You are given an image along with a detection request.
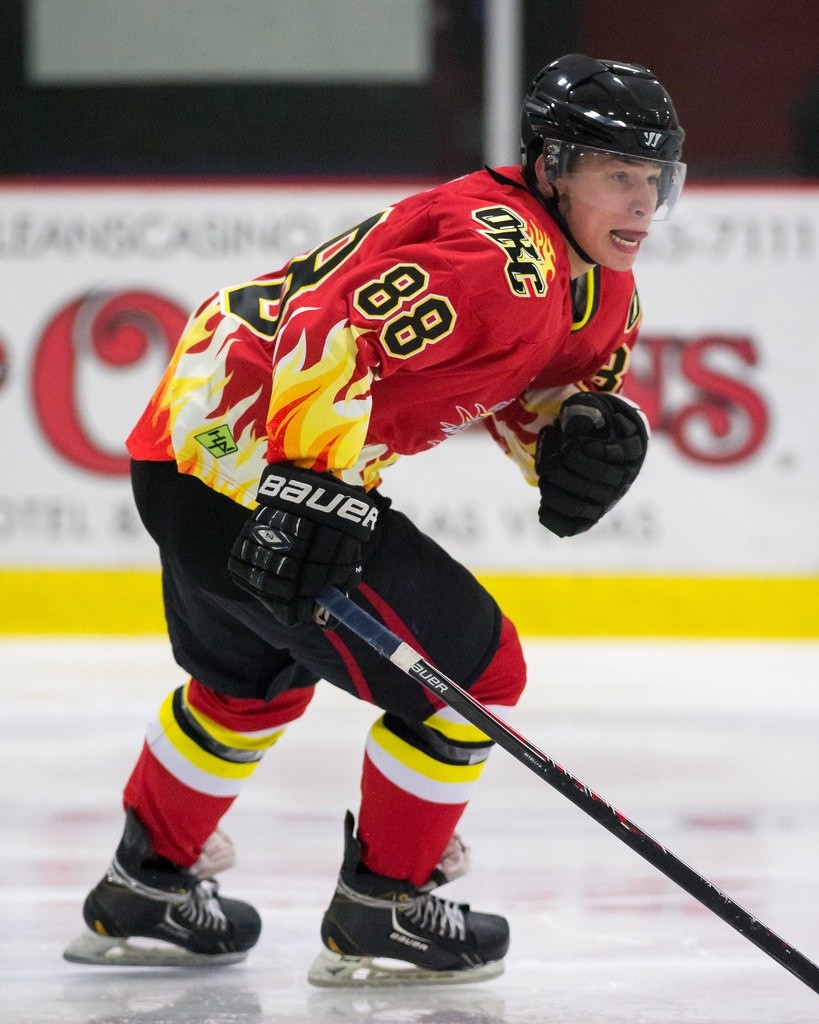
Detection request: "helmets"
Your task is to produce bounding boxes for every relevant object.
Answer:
[520,55,685,164]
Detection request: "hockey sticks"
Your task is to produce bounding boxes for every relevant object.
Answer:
[315,582,819,997]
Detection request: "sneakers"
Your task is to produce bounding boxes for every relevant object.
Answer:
[305,810,511,987]
[59,807,262,969]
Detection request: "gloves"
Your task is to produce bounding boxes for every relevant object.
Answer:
[225,465,392,629]
[534,390,651,539]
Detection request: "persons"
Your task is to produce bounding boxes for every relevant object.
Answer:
[63,50,691,986]
